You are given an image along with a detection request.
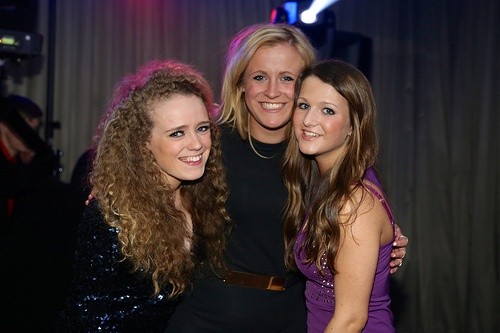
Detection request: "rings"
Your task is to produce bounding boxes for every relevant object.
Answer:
[395,259,403,267]
[400,235,408,246]
[399,249,405,259]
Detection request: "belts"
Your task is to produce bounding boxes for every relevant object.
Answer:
[198,262,291,292]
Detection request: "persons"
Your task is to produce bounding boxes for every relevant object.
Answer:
[85,25,409,333]
[0,57,236,333]
